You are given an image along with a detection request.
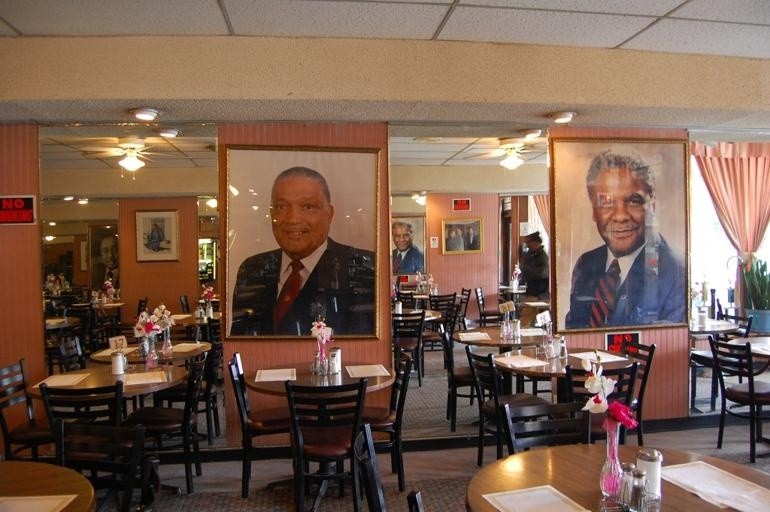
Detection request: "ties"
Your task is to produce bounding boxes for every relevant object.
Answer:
[270,260,306,336]
[589,259,622,328]
[106,271,114,288]
[393,252,402,275]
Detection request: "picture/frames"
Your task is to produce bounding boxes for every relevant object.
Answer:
[393,212,428,277]
[133,207,182,264]
[219,142,384,343]
[549,135,694,336]
[440,215,484,257]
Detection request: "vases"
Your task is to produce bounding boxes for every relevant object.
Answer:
[599,417,624,498]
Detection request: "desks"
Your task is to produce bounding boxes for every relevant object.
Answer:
[466,445,770,512]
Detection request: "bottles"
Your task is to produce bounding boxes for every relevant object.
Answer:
[532,344,542,357]
[637,446,663,505]
[416,271,434,292]
[541,359,570,373]
[193,306,205,319]
[498,318,521,338]
[395,301,403,314]
[545,341,554,358]
[616,463,648,512]
[699,328,706,331]
[313,348,341,375]
[92,294,107,304]
[112,375,125,385]
[698,310,707,328]
[314,376,342,387]
[195,318,206,323]
[427,283,438,294]
[47,273,69,294]
[500,339,521,345]
[391,277,399,296]
[557,340,568,359]
[110,352,129,375]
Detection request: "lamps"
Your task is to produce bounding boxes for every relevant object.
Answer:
[130,103,161,123]
[118,150,145,173]
[549,108,577,126]
[158,126,180,138]
[500,151,525,170]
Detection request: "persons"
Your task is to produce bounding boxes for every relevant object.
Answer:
[230,166,376,335]
[392,222,423,274]
[512,231,549,297]
[93,236,118,291]
[150,223,164,251]
[565,146,687,330]
[446,227,480,251]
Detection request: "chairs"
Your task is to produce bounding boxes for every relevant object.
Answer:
[0,254,225,510]
[228,349,423,511]
[392,279,458,387]
[441,285,657,467]
[689,289,770,463]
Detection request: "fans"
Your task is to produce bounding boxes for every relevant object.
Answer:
[455,134,550,161]
[78,137,175,163]
